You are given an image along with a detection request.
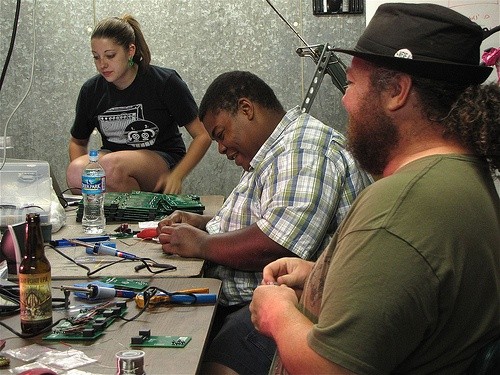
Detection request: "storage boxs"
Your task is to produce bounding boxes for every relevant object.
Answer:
[0,163,52,224]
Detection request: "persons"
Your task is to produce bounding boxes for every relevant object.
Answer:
[248,3,500,375]
[156,71,374,375]
[66,16,212,196]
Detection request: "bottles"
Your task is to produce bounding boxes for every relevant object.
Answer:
[19,213,52,333]
[82,150,105,233]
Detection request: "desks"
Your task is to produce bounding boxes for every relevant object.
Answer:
[0,278,224,375]
[0,195,226,276]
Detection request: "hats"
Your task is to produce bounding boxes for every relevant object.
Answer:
[329,3,494,85]
